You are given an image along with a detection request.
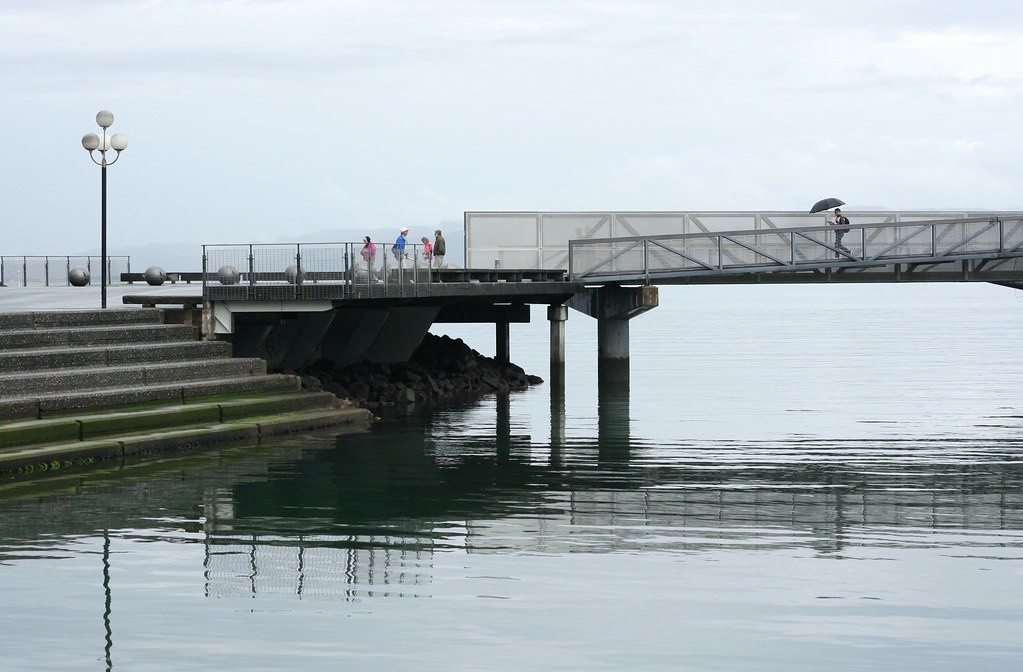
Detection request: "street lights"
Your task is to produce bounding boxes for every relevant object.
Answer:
[82,109,128,309]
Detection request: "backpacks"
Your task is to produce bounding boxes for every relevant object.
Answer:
[840,217,850,232]
[392,244,399,254]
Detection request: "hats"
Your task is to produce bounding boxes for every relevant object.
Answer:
[401,228,410,233]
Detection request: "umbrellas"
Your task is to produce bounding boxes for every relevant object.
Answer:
[809,196,845,224]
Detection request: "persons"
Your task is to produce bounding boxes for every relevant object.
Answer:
[432,229,445,270]
[421,236,433,269]
[826,208,853,260]
[390,227,412,282]
[361,236,379,281]
